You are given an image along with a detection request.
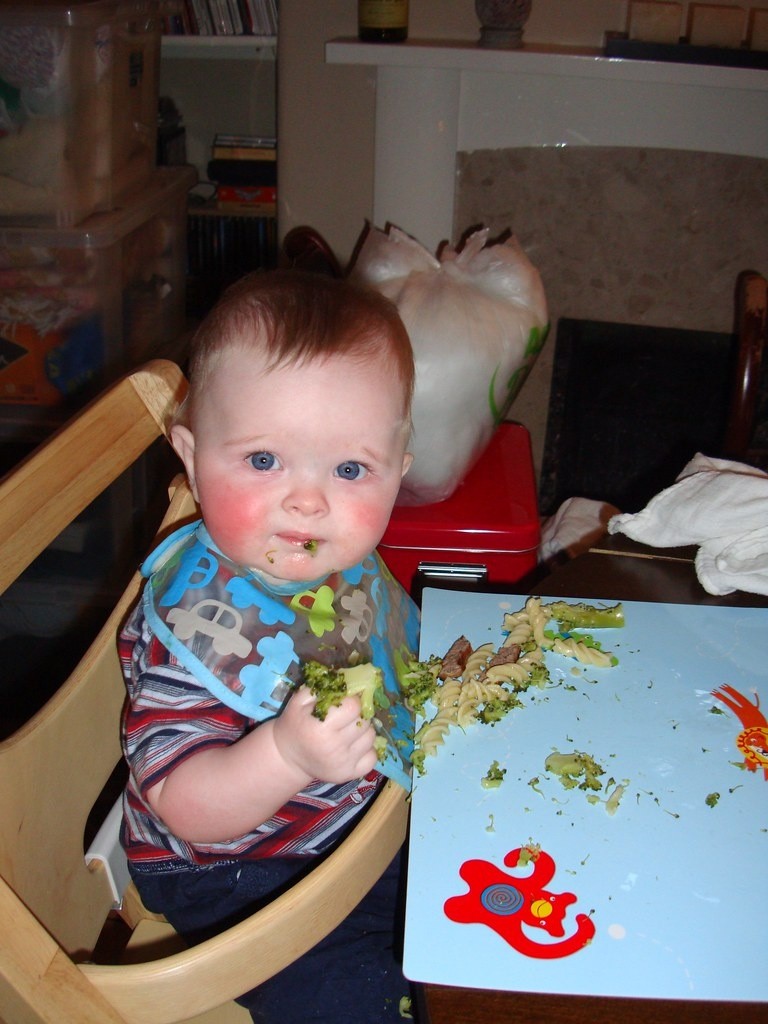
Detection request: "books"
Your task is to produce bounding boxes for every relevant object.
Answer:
[206,133,278,218]
[160,0,278,36]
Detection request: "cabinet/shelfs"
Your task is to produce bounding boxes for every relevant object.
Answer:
[158,34,279,216]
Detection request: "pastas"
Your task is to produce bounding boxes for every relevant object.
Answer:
[420,596,613,757]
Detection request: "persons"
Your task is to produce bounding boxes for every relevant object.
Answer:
[114,253,424,1024]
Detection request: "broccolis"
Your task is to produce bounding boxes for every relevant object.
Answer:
[303,539,626,793]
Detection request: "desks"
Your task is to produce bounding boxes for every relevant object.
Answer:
[1,165,200,509]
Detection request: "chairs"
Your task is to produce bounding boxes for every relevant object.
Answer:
[0,358,411,1024]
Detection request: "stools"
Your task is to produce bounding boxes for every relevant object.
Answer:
[0,391,143,577]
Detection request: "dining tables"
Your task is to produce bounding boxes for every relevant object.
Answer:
[402,531,768,1024]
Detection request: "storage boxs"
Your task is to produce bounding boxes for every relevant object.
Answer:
[0,268,107,406]
[0,0,157,215]
[377,422,541,611]
[212,138,277,185]
[215,184,278,216]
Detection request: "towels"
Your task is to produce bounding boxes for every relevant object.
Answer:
[536,452,768,598]
